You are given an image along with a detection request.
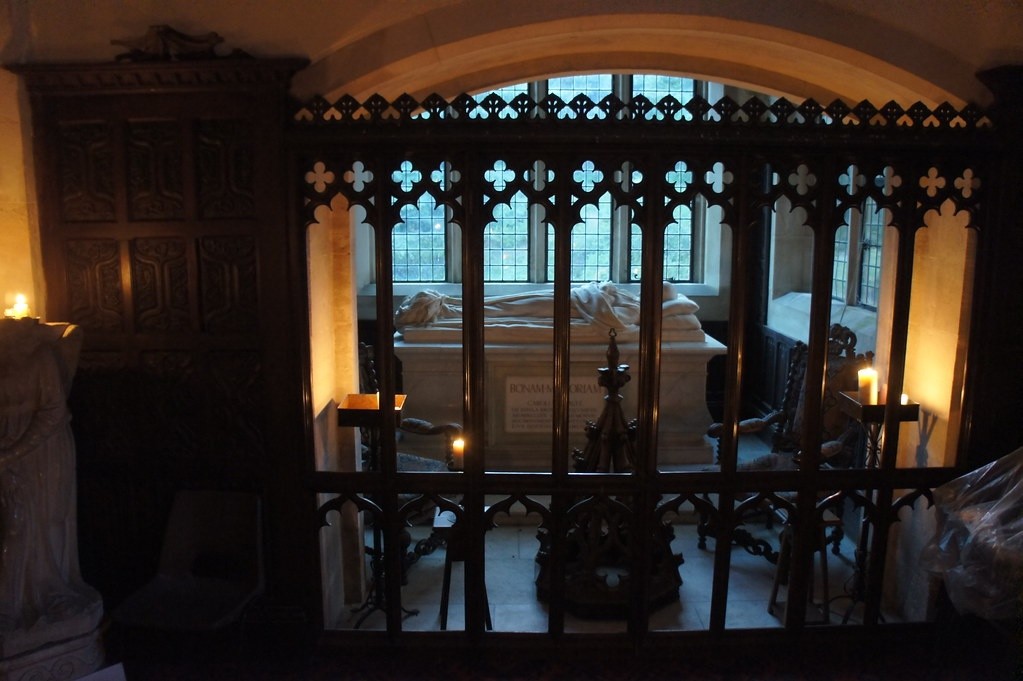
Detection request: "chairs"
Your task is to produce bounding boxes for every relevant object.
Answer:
[696,324,874,586]
[358,340,464,609]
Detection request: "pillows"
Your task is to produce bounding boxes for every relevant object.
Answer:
[661,292,700,317]
[661,314,702,330]
[662,329,706,342]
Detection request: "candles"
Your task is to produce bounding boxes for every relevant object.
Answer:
[13,294,28,316]
[857,368,878,404]
